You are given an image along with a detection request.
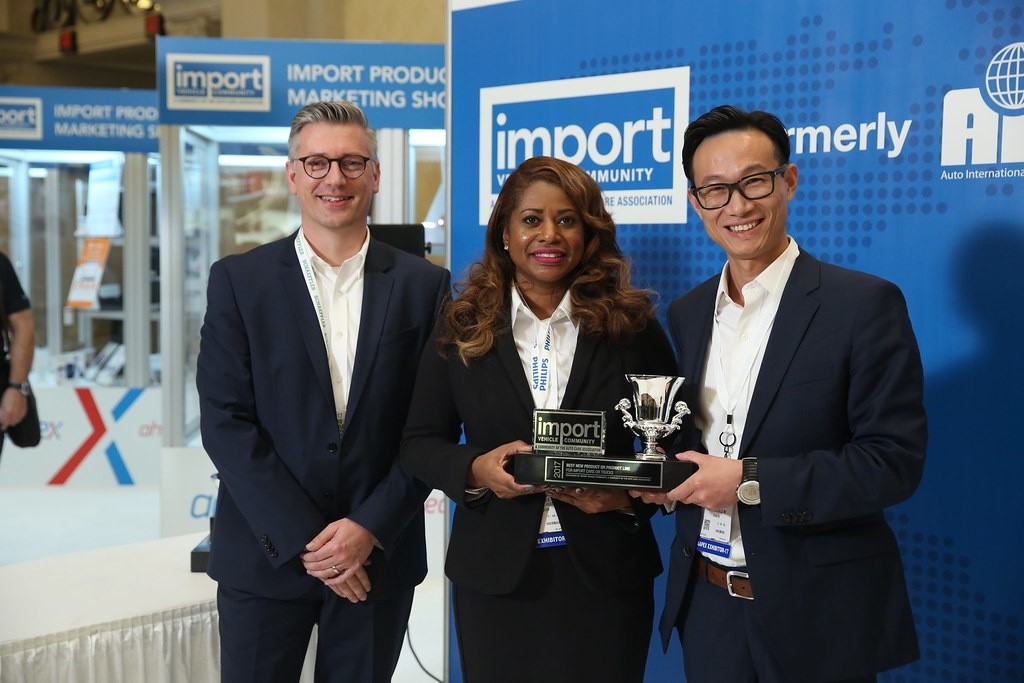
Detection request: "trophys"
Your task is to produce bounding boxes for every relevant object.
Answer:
[513,374,694,493]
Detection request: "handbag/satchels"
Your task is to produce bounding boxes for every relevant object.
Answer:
[4,319,41,447]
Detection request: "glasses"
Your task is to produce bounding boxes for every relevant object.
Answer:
[291,155,377,179]
[691,163,788,210]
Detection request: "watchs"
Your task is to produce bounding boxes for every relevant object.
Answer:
[736,456,761,506]
[7,383,30,396]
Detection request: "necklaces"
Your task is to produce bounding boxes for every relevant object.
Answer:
[515,282,530,310]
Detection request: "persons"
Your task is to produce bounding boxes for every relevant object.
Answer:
[0,251,35,455]
[629,106,928,683]
[397,156,678,683]
[197,100,452,683]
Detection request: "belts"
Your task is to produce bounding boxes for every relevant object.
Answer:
[692,556,756,600]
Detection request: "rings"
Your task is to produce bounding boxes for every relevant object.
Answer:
[333,566,340,574]
